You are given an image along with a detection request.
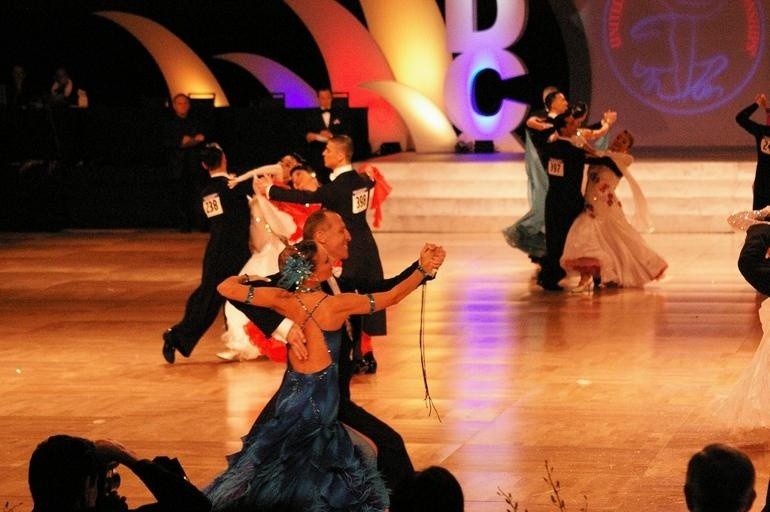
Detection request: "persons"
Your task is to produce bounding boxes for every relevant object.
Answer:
[526,86,635,291]
[301,89,348,179]
[228,211,447,484]
[161,95,211,235]
[163,135,388,375]
[393,466,463,512]
[205,240,439,511]
[684,443,757,512]
[727,93,770,297]
[7,65,31,105]
[51,61,74,108]
[28,435,212,512]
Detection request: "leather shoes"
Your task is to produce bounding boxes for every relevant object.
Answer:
[162,328,175,363]
[216,349,243,362]
[350,356,377,374]
[537,278,564,291]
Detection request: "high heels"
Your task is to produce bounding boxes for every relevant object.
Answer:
[572,274,594,292]
